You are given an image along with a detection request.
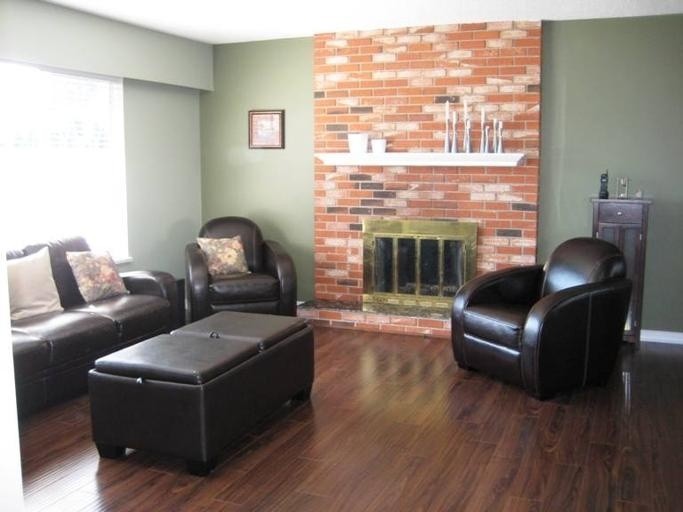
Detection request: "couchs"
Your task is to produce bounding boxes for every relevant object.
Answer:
[452,237,634,401]
[6,237,179,420]
[185,217,297,324]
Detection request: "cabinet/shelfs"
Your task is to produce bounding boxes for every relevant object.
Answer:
[589,197,653,351]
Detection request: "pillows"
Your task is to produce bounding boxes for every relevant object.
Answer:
[7,246,64,322]
[196,234,252,280]
[65,252,130,302]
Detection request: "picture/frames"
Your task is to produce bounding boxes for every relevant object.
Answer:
[248,109,285,150]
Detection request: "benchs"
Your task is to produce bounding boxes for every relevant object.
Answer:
[88,310,315,478]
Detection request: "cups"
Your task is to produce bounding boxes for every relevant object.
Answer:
[347,133,367,155]
[370,139,385,160]
